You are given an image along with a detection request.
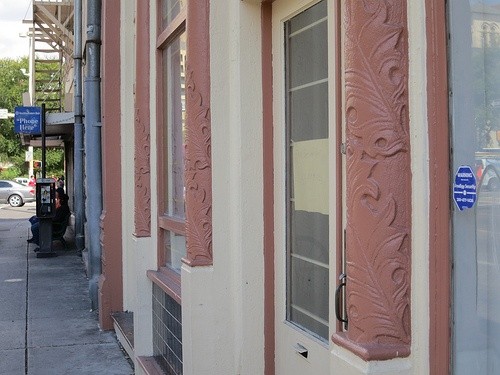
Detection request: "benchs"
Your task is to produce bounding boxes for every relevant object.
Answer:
[33,212,71,253]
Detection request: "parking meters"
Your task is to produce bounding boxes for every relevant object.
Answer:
[34,178,57,257]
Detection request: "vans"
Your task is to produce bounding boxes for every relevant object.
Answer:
[14,178,36,187]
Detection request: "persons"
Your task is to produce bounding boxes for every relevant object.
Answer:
[31,194,71,252]
[27,175,65,243]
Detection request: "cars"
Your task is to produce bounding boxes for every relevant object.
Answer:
[0,179,36,207]
